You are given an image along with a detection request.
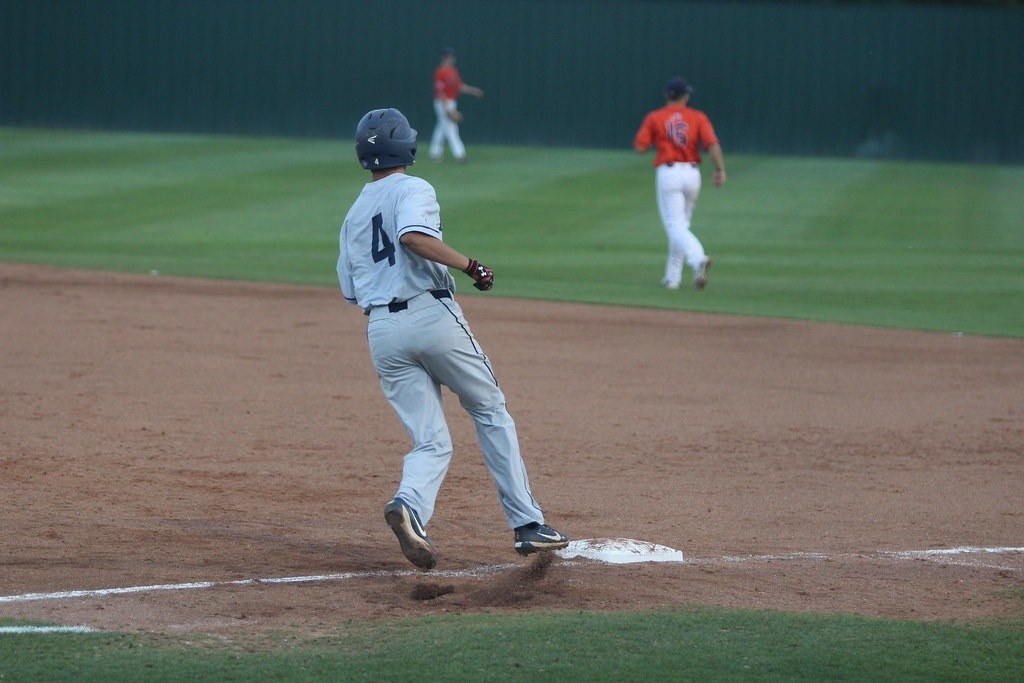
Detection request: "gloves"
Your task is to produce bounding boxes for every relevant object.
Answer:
[463,258,495,291]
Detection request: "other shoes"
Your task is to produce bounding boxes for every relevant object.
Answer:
[696,256,710,288]
[663,279,680,290]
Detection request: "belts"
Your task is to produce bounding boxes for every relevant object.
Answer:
[368,289,452,313]
[668,163,699,168]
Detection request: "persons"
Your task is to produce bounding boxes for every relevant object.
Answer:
[634,79,726,292]
[336,107,571,571]
[428,46,484,166]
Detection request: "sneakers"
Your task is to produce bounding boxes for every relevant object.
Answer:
[514,521,569,554]
[384,498,437,569]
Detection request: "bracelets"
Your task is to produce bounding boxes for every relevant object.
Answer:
[715,168,725,171]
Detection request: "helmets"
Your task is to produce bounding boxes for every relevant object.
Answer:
[355,108,418,169]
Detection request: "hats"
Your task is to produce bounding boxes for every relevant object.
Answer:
[667,77,693,95]
